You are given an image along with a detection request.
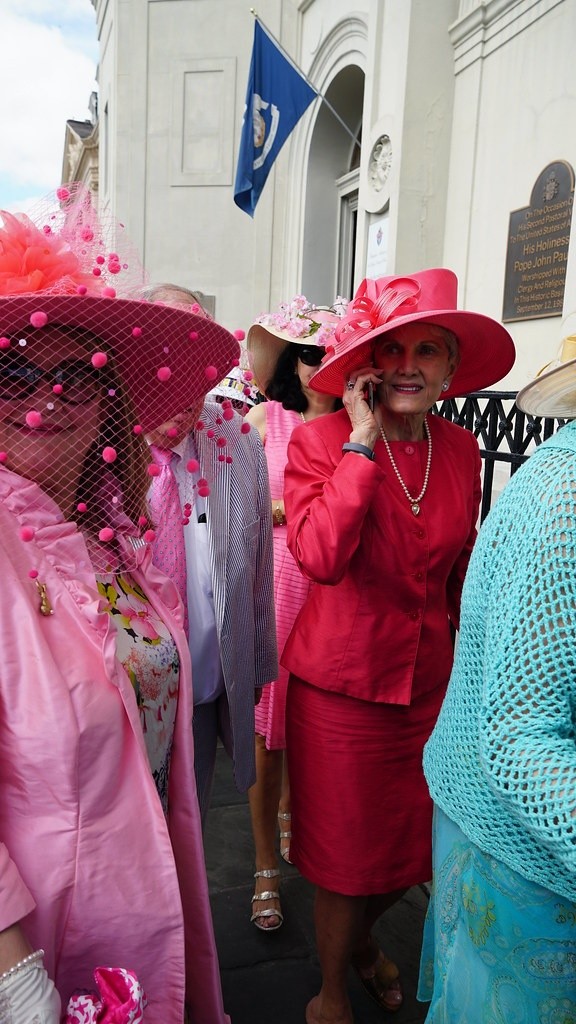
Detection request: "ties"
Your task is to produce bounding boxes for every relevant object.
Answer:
[145,444,190,641]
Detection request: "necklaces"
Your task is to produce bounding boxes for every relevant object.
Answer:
[379,414,433,517]
[300,411,307,423]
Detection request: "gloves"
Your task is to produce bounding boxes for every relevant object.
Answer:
[0,958,62,1024]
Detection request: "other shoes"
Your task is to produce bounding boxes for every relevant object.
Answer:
[349,936,408,1014]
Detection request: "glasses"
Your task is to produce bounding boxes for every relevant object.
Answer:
[215,395,241,406]
[0,350,103,405]
[292,346,325,366]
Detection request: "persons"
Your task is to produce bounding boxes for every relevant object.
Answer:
[205,359,258,417]
[279,266,518,1024]
[236,299,349,935]
[126,283,268,806]
[419,337,576,1024]
[1,181,238,1024]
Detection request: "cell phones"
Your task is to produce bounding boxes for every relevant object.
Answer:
[367,381,374,413]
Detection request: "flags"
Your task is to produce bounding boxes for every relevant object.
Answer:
[233,14,320,221]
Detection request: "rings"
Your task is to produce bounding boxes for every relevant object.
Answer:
[346,380,355,390]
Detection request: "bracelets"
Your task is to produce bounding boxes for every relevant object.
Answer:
[0,947,46,980]
[342,443,376,461]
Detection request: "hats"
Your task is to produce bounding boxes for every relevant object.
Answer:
[0,182,241,434]
[308,268,517,401]
[515,335,576,419]
[245,293,350,401]
[206,328,259,406]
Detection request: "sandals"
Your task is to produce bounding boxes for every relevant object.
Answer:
[276,811,293,865]
[249,869,284,930]
